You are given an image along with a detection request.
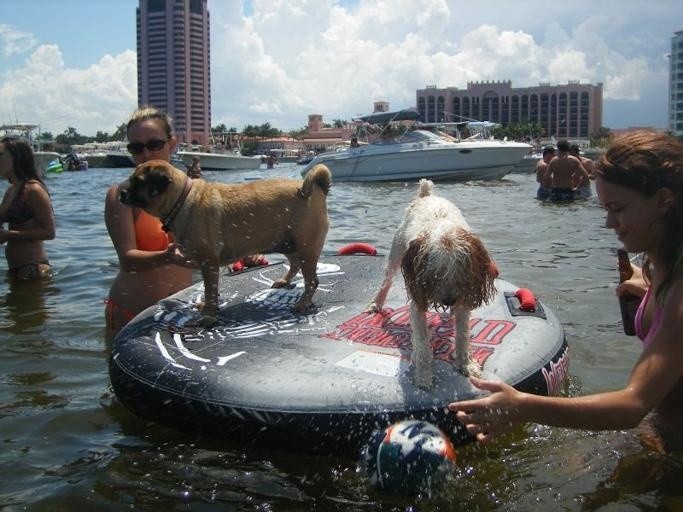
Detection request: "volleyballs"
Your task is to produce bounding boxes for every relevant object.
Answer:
[364,420,457,498]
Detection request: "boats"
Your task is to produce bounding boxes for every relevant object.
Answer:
[0,123,61,178]
[299,104,534,184]
[174,127,265,173]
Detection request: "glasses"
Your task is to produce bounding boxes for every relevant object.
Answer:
[126,139,168,154]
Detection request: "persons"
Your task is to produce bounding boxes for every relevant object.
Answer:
[297,148,301,157]
[267,153,279,169]
[446,128,683,453]
[349,133,360,150]
[102,106,269,337]
[535,145,556,201]
[1,134,58,282]
[568,142,599,201]
[300,148,305,157]
[63,152,89,172]
[544,139,589,204]
[186,156,204,180]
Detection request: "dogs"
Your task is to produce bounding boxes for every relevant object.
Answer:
[117,159,332,317]
[369,178,499,392]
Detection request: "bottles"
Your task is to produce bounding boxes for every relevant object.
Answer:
[616,248,643,337]
